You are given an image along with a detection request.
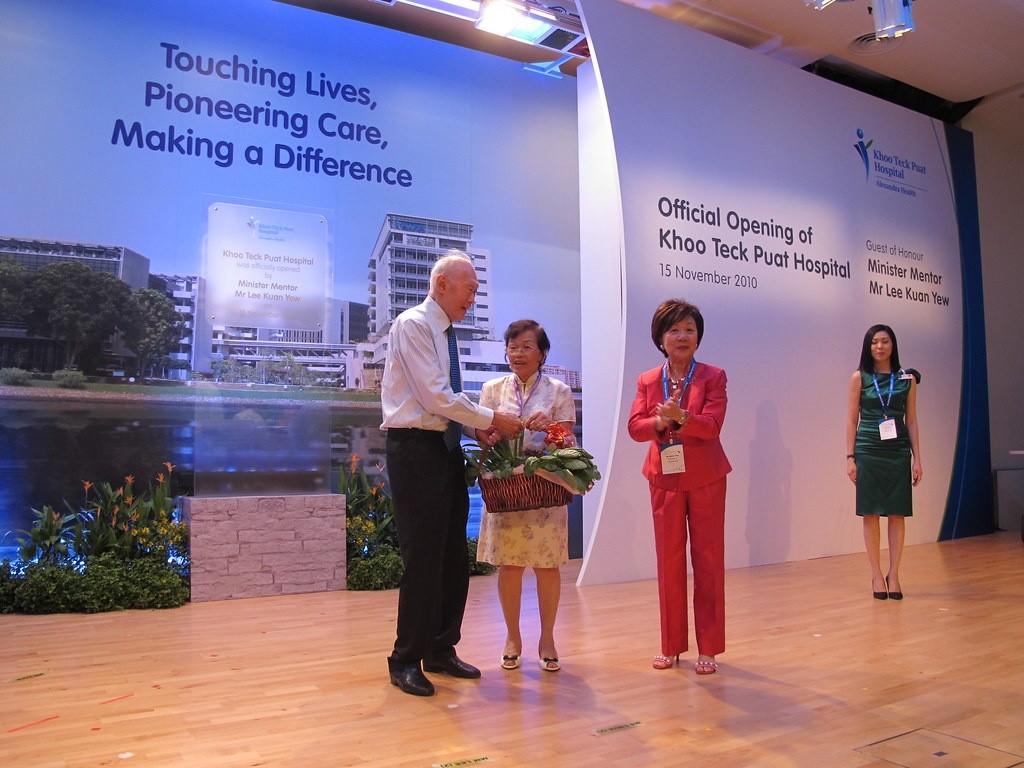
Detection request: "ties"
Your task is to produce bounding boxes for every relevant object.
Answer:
[443,325,462,452]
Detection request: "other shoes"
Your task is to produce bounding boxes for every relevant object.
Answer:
[540,657,561,671]
[501,653,523,669]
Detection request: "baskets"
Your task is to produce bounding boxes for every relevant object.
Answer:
[478,422,573,513]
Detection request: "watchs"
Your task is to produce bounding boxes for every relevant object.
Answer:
[677,410,686,425]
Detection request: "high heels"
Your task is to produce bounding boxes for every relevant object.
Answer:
[696,662,717,674]
[872,579,888,599]
[653,654,680,669]
[885,576,903,600]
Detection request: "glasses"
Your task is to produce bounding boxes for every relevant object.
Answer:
[505,344,540,355]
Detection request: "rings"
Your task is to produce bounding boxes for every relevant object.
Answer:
[539,422,542,425]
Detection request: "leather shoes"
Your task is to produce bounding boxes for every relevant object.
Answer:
[424,653,481,679]
[389,666,434,697]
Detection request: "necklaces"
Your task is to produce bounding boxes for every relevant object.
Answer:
[514,371,542,418]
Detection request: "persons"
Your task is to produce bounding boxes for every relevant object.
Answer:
[847,324,922,600]
[379,249,526,696]
[477,320,575,670]
[628,299,732,674]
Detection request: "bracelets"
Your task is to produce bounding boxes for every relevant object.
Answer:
[847,455,854,459]
